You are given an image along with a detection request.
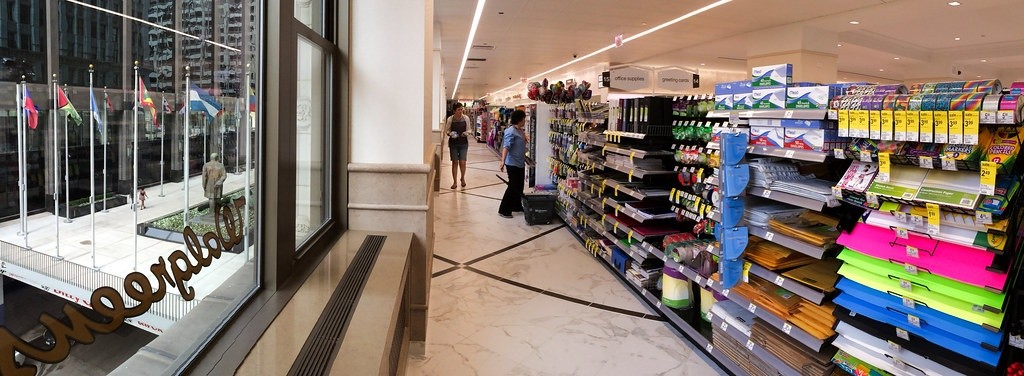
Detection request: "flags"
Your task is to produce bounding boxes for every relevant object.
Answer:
[15,77,257,132]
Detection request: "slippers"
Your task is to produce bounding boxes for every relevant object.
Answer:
[460,179,466,187]
[451,184,457,189]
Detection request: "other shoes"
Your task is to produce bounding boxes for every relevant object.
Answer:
[500,212,513,218]
[513,207,524,212]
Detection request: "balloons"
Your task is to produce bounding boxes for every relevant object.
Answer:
[527,78,592,104]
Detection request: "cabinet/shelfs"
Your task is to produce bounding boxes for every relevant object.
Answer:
[463,103,1024,376]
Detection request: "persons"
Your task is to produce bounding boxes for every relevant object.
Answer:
[446,102,472,189]
[139,187,148,210]
[129,187,134,209]
[498,110,532,218]
[203,152,228,217]
